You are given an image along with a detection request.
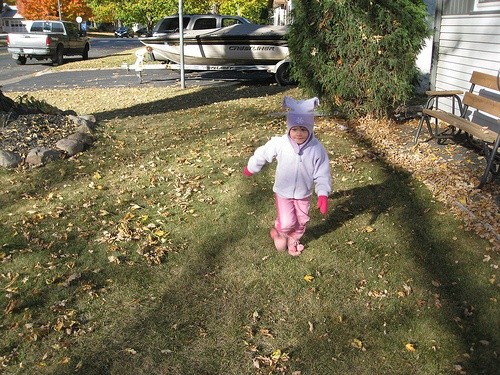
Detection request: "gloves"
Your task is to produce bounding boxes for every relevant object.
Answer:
[316,195,329,214]
[243,167,252,176]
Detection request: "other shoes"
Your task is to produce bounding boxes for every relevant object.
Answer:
[287,237,304,256]
[271,228,288,252]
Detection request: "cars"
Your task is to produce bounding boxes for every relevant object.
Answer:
[113,26,134,38]
[136,28,155,36]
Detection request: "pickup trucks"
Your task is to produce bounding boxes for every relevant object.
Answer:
[6,20,90,66]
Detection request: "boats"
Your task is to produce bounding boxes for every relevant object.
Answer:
[139,23,316,65]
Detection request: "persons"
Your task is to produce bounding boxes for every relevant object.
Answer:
[243,97,332,256]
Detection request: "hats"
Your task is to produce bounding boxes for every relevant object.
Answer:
[283,96,320,154]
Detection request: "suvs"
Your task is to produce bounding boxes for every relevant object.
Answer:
[148,14,254,73]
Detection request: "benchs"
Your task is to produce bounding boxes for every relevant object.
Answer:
[413,70,500,190]
